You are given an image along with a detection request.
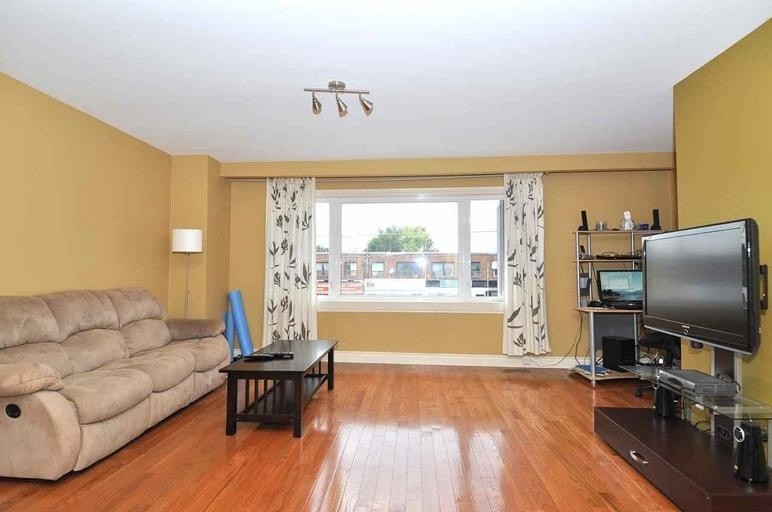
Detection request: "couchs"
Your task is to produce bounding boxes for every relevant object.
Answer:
[0,287,231,482]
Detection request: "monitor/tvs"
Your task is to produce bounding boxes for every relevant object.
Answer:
[597,269,642,303]
[643,219,761,355]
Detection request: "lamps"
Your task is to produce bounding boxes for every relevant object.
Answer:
[304,82,373,114]
[171,229,203,320]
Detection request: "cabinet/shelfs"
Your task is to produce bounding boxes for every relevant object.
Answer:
[573,230,661,308]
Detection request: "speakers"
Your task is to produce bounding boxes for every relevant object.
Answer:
[578,210,588,230]
[651,209,661,230]
[602,335,636,373]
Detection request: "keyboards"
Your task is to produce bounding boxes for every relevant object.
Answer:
[615,303,643,310]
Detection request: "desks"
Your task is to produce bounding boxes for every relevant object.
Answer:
[574,308,643,388]
[594,364,772,512]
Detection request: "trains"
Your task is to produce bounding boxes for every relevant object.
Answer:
[315,252,497,296]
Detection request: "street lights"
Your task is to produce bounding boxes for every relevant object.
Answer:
[168,226,205,316]
